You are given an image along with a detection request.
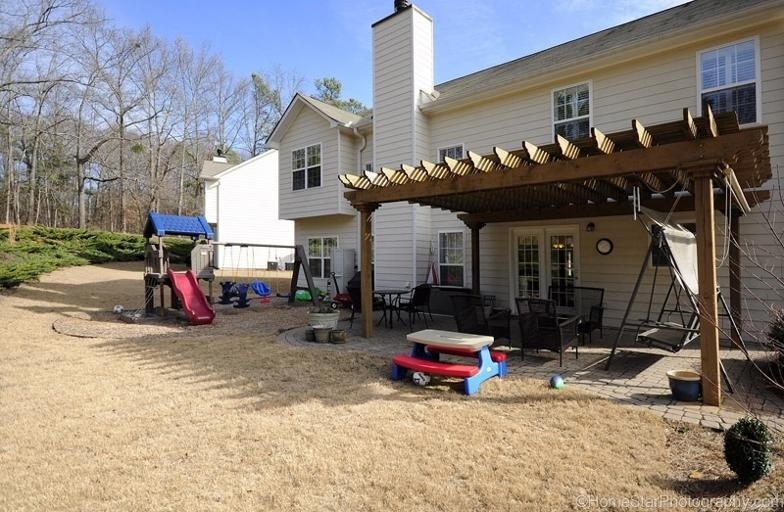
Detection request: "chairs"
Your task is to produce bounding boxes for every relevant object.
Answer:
[514,295,582,371]
[390,283,437,329]
[546,285,605,343]
[346,271,387,331]
[450,290,514,348]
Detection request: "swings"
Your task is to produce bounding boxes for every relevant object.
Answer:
[252,246,272,304]
[234,245,252,308]
[636,230,701,352]
[219,245,238,305]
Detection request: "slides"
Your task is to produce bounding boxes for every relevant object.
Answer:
[167,268,216,325]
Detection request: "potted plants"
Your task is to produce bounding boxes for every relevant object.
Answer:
[724,417,774,482]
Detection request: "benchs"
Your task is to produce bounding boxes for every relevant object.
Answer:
[422,342,508,364]
[634,291,722,355]
[390,355,480,399]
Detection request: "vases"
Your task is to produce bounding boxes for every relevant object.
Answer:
[665,370,701,402]
[304,308,345,343]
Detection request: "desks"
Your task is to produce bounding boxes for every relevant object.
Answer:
[405,326,495,354]
[374,289,412,327]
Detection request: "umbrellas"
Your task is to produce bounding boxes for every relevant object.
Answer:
[425,260,440,285]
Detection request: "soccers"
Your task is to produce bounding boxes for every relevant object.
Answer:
[412,371,431,386]
[114,305,124,314]
